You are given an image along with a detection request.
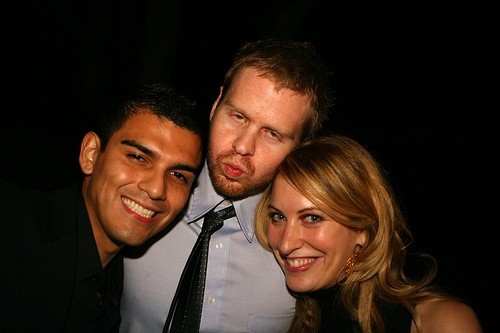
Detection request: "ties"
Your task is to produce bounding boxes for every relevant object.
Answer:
[162,206,236,333]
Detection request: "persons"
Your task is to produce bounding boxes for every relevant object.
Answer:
[115,34,338,333]
[253,130,482,333]
[0,79,210,333]
